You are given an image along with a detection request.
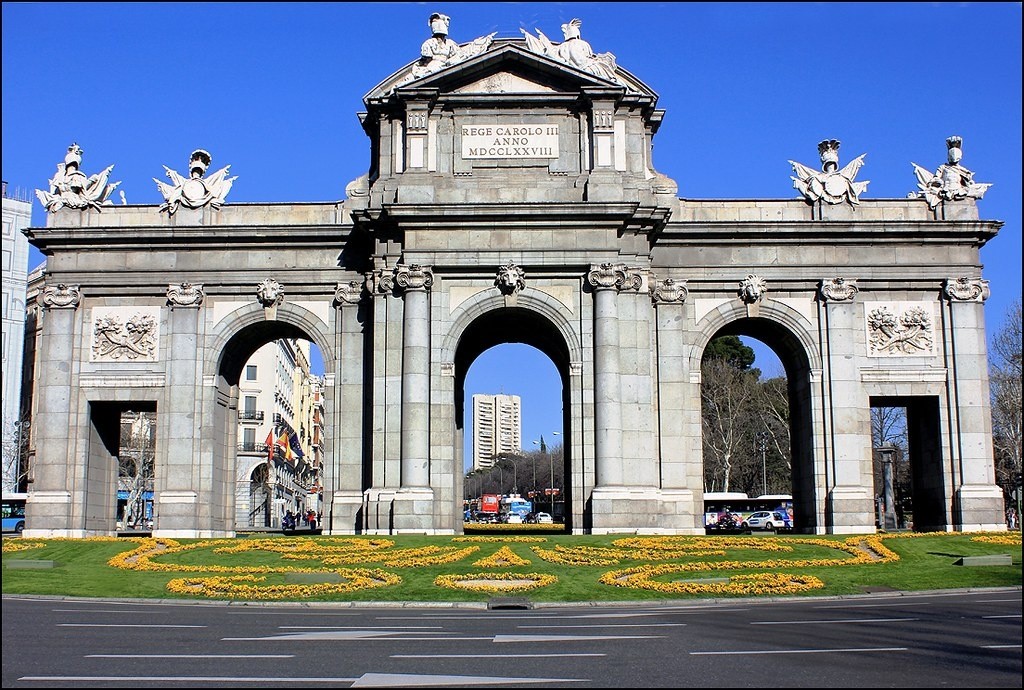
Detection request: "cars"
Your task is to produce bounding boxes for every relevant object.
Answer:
[462,494,534,524]
[740,511,786,530]
[531,511,553,524]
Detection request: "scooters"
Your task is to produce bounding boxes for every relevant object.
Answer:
[281,516,296,531]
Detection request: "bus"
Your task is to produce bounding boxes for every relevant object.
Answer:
[703,492,794,528]
[2,491,154,534]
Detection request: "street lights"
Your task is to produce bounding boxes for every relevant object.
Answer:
[501,457,517,497]
[532,440,554,519]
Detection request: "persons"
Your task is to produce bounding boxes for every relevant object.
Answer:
[465,509,479,522]
[283,509,321,528]
[725,509,732,519]
[1006,508,1018,529]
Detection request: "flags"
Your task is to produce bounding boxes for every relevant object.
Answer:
[265,429,273,460]
[276,429,292,461]
[289,433,305,457]
